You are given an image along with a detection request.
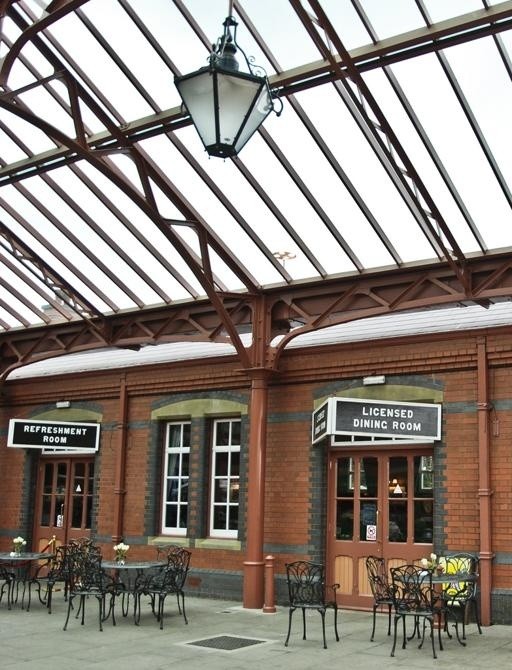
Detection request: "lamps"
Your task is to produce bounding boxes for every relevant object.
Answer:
[172,0,285,164]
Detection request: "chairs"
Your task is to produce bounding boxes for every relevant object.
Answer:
[0,535,193,634]
[364,552,487,660]
[283,559,341,650]
